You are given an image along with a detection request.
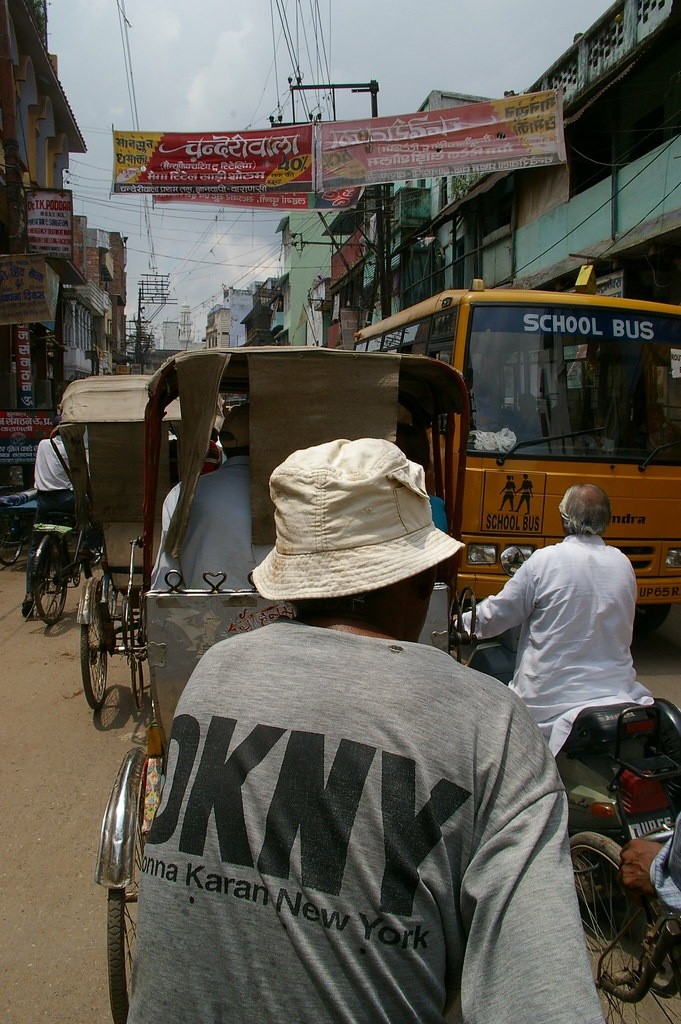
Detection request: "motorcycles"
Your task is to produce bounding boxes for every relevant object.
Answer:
[432,546,680,914]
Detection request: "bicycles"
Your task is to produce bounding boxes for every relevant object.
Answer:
[0,483,34,566]
[29,512,104,625]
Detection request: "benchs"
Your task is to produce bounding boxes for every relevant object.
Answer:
[142,570,449,757]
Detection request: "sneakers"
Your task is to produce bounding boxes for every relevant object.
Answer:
[20,591,33,620]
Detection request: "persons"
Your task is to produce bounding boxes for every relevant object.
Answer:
[396,424,448,536]
[22,415,84,620]
[454,482,654,757]
[146,403,275,590]
[111,438,614,1024]
[467,349,615,454]
[619,811,681,912]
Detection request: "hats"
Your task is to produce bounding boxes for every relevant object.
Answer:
[217,405,249,448]
[252,438,464,600]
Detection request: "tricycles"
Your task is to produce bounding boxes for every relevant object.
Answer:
[49,344,222,712]
[93,349,467,1022]
[567,831,681,1024]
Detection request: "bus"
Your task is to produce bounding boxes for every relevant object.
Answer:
[352,263,681,639]
